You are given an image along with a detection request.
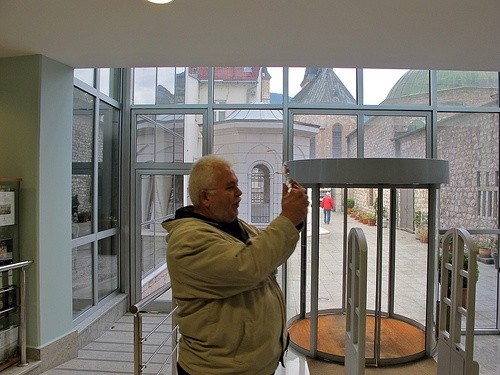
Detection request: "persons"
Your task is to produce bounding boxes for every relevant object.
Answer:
[320,192,334,224]
[161,154,308,375]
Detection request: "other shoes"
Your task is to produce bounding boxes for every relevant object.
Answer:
[324,222,330,224]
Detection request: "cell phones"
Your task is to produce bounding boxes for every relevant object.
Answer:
[283,161,293,188]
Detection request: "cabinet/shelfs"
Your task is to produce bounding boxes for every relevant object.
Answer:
[0,177,22,372]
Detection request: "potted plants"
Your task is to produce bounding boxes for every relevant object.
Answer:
[344,198,388,227]
[437,252,481,309]
[479,241,492,256]
[420,225,428,243]
[492,235,500,270]
[413,212,423,240]
